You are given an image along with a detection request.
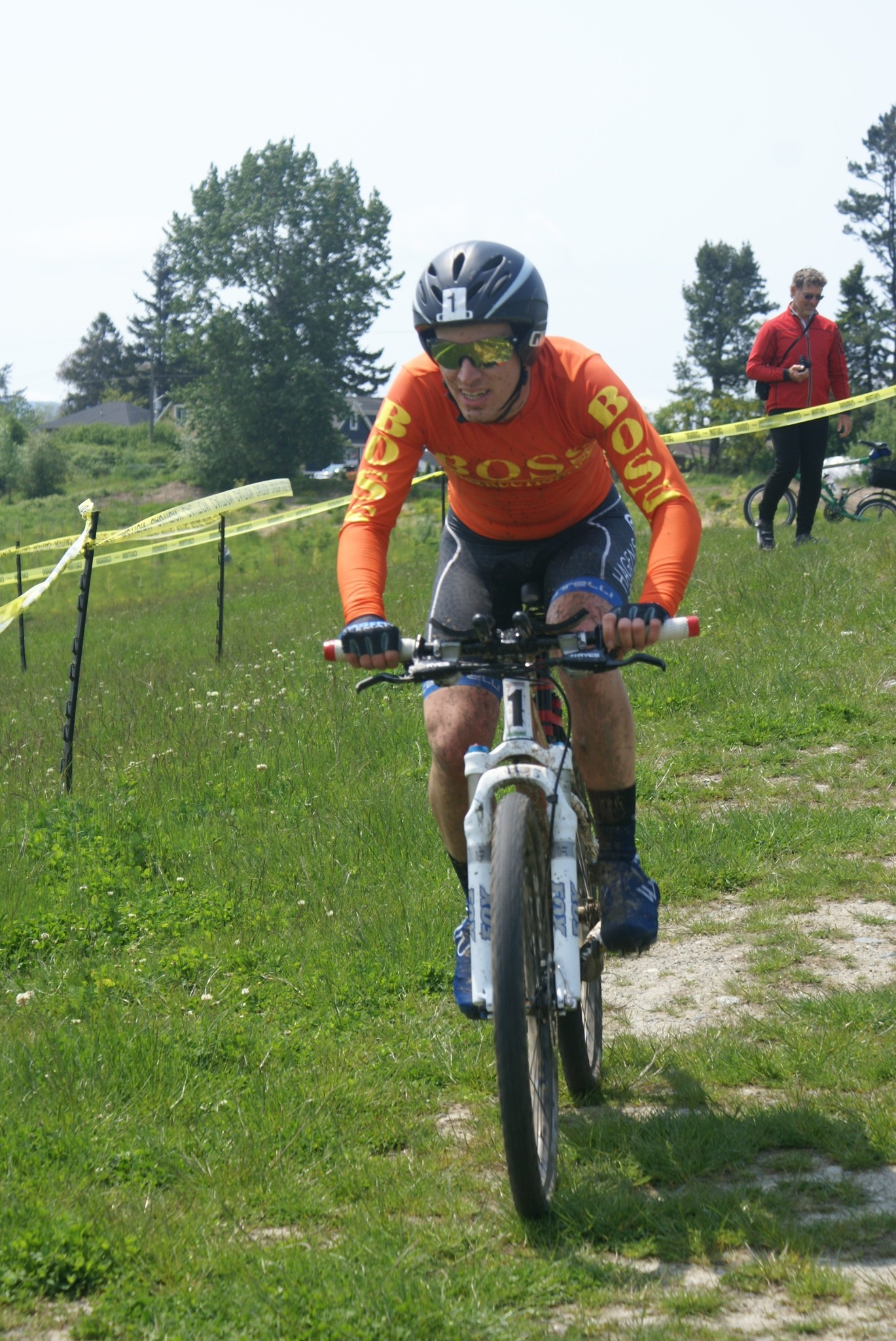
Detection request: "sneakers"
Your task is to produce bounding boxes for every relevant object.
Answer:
[795,533,817,546]
[596,852,661,953]
[755,520,775,550]
[453,916,488,1019]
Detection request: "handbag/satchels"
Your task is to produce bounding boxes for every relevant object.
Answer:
[868,466,896,490]
[755,381,768,400]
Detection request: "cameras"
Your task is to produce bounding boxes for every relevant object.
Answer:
[798,356,811,372]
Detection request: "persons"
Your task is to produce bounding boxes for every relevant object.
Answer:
[335,240,703,1023]
[744,268,854,551]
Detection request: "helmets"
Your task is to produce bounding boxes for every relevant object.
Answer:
[413,240,548,364]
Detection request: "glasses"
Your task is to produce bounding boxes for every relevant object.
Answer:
[424,335,516,369]
[797,287,824,300]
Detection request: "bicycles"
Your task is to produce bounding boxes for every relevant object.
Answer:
[320,573,703,1221]
[742,438,896,530]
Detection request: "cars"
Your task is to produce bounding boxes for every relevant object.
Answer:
[417,448,445,479]
[310,463,357,481]
